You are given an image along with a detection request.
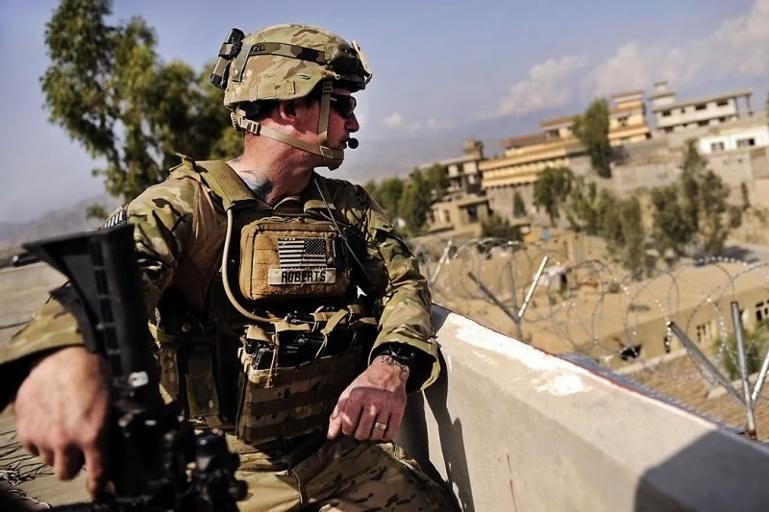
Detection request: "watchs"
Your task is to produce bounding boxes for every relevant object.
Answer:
[372,344,418,368]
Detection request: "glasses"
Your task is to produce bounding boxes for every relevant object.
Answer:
[311,90,357,118]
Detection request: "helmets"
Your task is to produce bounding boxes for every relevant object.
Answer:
[213,23,366,109]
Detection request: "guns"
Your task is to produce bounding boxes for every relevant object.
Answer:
[21,222,249,512]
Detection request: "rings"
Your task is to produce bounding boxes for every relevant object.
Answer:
[375,422,388,429]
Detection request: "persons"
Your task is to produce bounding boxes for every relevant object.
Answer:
[0,24,464,512]
